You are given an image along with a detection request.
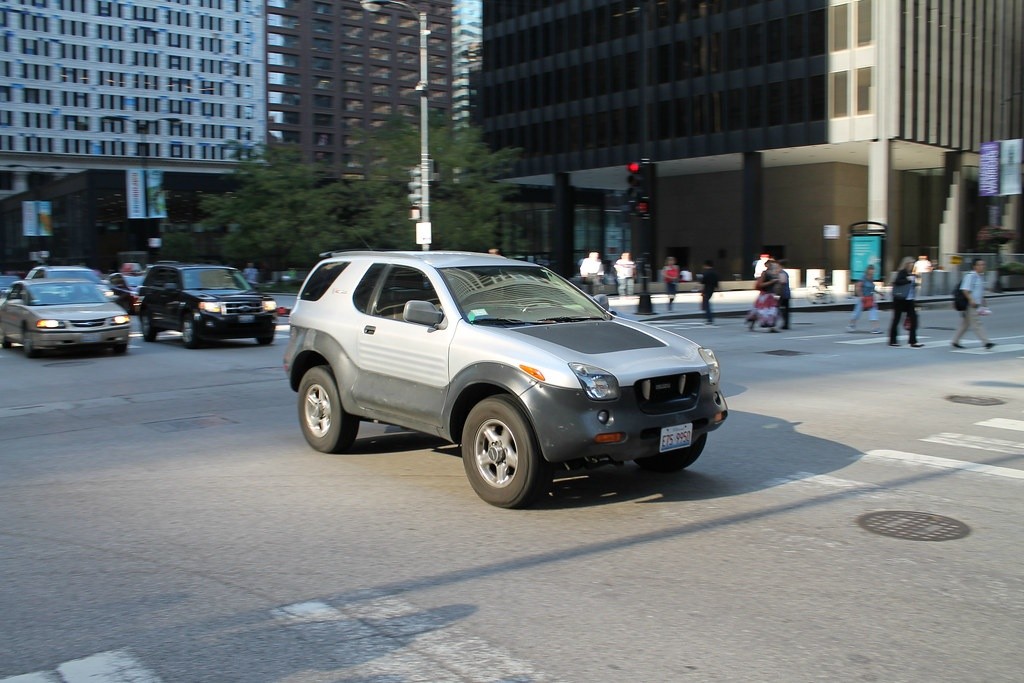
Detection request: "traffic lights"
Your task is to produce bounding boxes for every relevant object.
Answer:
[625,158,649,206]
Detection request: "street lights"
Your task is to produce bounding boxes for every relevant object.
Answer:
[357,0,431,251]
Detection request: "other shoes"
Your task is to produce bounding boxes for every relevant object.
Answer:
[844,326,856,333]
[951,342,964,350]
[870,329,882,334]
[911,343,925,349]
[985,343,997,350]
[889,343,903,346]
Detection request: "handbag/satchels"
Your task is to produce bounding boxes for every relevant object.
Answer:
[892,281,911,299]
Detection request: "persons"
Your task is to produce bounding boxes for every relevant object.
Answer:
[243,263,259,286]
[580,251,605,294]
[847,265,884,335]
[888,255,924,347]
[952,258,995,349]
[662,256,680,311]
[912,254,942,295]
[699,260,719,325]
[678,269,693,282]
[746,261,791,331]
[614,253,637,295]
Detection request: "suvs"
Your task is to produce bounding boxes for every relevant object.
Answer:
[137,260,281,347]
[283,250,730,510]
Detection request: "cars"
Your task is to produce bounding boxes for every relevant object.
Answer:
[0,276,23,308]
[21,265,119,302]
[0,277,132,360]
[106,270,145,314]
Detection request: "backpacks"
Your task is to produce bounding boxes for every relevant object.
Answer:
[952,279,969,312]
